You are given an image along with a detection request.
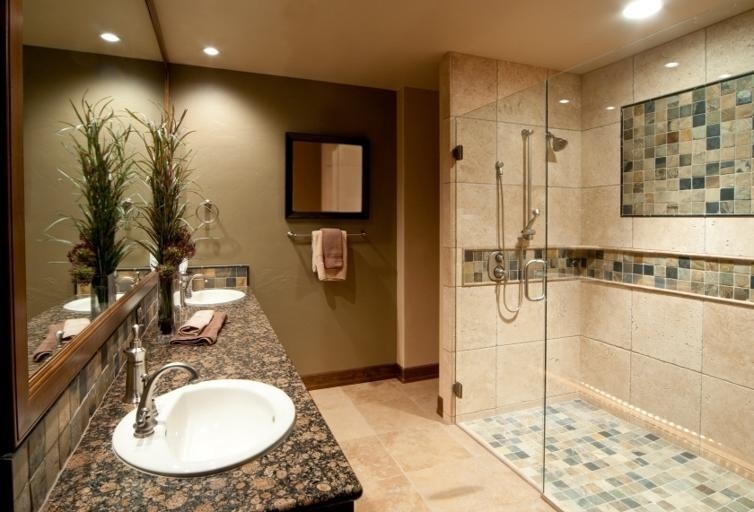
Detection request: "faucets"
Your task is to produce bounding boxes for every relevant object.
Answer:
[136,360,196,421]
[178,273,203,298]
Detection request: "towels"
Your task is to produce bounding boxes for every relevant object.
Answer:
[170,310,226,347]
[30,319,87,370]
[311,227,348,282]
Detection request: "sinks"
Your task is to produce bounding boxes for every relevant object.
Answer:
[63,292,126,314]
[111,379,297,474]
[173,288,247,306]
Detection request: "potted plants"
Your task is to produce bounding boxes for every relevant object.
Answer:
[46,88,142,322]
[126,107,204,334]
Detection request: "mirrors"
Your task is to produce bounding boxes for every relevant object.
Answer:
[285,130,370,220]
[2,0,173,453]
